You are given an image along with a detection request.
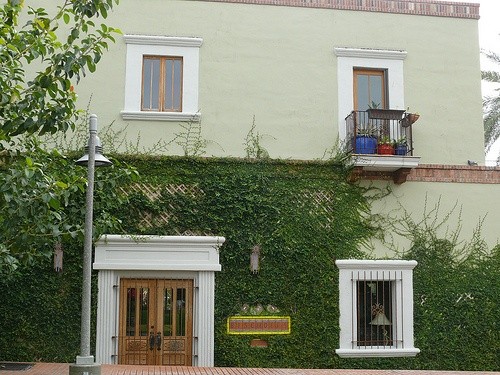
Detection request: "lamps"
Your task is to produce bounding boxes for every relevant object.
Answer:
[368,312,393,345]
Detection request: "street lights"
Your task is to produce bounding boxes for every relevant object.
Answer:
[72,114,113,364]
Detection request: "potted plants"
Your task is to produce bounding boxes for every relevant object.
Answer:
[356,124,408,156]
